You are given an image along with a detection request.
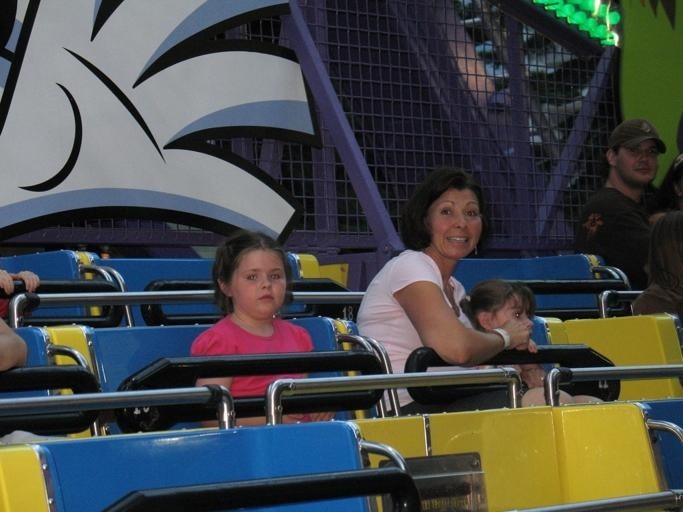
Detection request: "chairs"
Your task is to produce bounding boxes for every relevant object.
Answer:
[0,247,683,512]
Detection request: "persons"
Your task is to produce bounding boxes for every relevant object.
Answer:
[0,314,26,372]
[628,208,682,329]
[0,267,42,328]
[574,117,670,292]
[188,229,340,428]
[354,163,608,417]
[652,152,682,210]
[458,278,549,390]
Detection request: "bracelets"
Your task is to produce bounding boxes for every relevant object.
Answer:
[489,325,511,353]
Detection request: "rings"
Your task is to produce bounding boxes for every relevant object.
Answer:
[538,374,545,381]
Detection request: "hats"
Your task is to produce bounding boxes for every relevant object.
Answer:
[607,118,667,154]
